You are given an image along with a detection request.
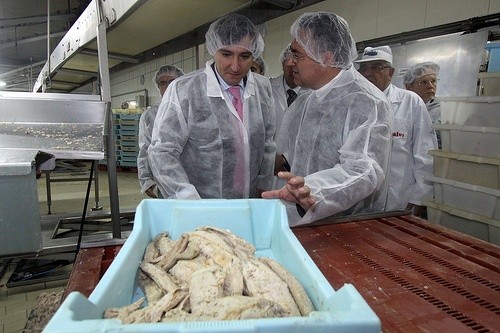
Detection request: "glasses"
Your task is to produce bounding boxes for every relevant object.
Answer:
[415,78,440,86]
[157,80,168,89]
[356,65,390,74]
[289,49,307,62]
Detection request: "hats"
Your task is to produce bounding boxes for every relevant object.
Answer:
[353,45,393,64]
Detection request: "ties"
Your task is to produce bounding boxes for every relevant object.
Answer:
[286,89,297,108]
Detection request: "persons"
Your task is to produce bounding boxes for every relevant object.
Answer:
[137,46,442,212]
[147,15,276,199]
[262,11,391,227]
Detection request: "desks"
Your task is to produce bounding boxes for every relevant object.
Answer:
[58,212,500,333]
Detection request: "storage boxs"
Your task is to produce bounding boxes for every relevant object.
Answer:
[425,176,500,221]
[433,124,500,155]
[485,42,500,72]
[112,108,146,168]
[427,150,500,191]
[422,199,500,246]
[435,96,500,129]
[38,199,382,333]
[478,72,500,96]
